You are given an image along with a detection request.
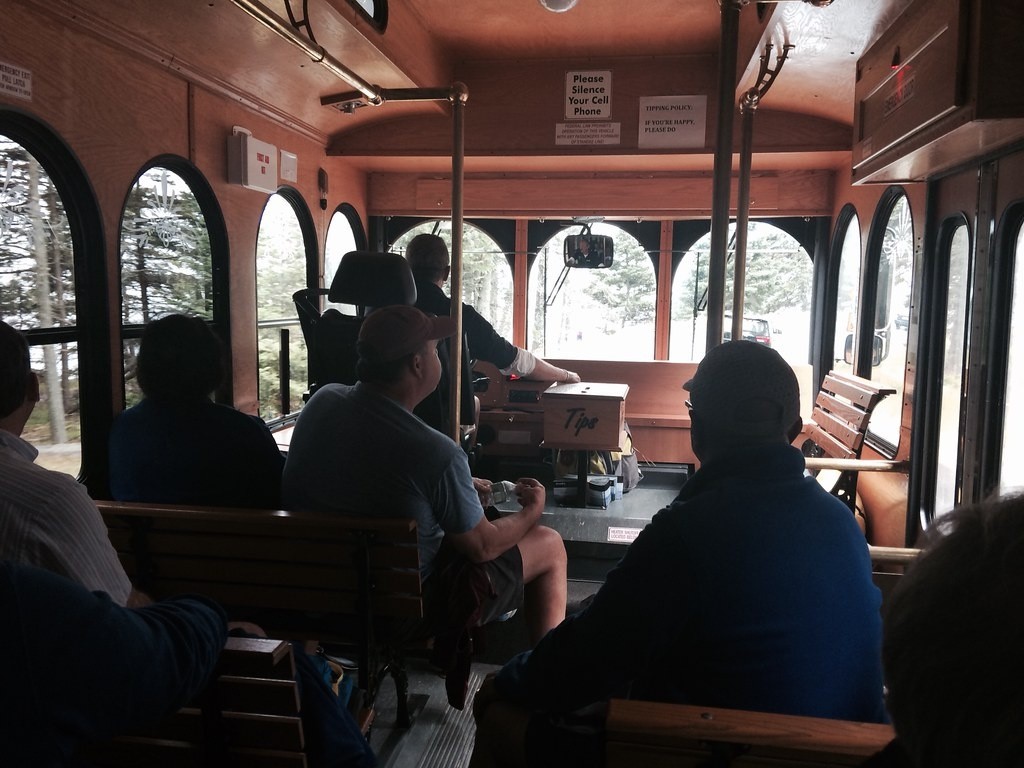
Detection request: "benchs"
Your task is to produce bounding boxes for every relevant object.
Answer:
[604,547,940,768]
[0,497,502,768]
[796,366,898,516]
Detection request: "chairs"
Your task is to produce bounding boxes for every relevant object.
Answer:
[307,250,476,462]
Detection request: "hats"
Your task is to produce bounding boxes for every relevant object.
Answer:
[357,305,458,362]
[684,340,800,436]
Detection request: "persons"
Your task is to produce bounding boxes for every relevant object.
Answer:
[0,558,230,768]
[467,341,886,768]
[407,234,580,455]
[854,491,1024,768]
[0,322,265,637]
[280,304,569,643]
[566,238,606,267]
[102,313,287,511]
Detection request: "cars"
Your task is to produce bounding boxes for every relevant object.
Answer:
[724,314,782,350]
[895,308,909,330]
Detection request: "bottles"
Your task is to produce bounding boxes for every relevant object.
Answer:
[477,480,517,508]
